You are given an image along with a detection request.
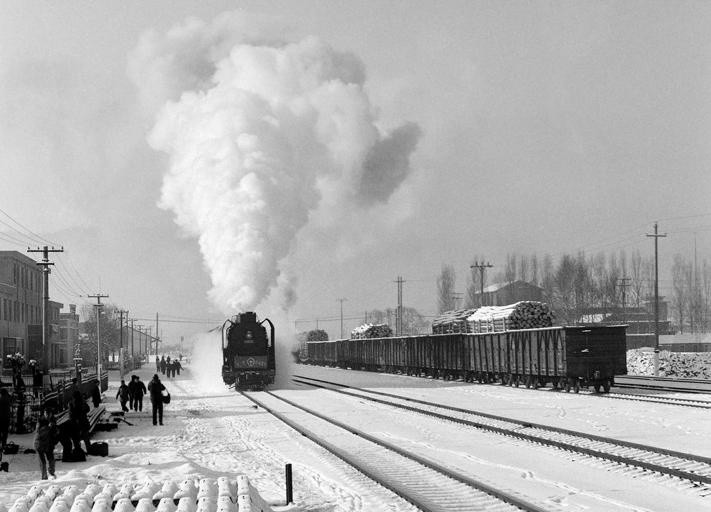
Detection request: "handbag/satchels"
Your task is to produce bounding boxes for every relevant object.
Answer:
[4,443,18,455]
[162,394,170,404]
[92,441,108,455]
[63,447,86,462]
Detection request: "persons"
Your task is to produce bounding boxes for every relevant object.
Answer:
[0,355,185,479]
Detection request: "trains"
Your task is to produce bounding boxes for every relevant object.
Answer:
[288,323,631,394]
[187,311,275,394]
